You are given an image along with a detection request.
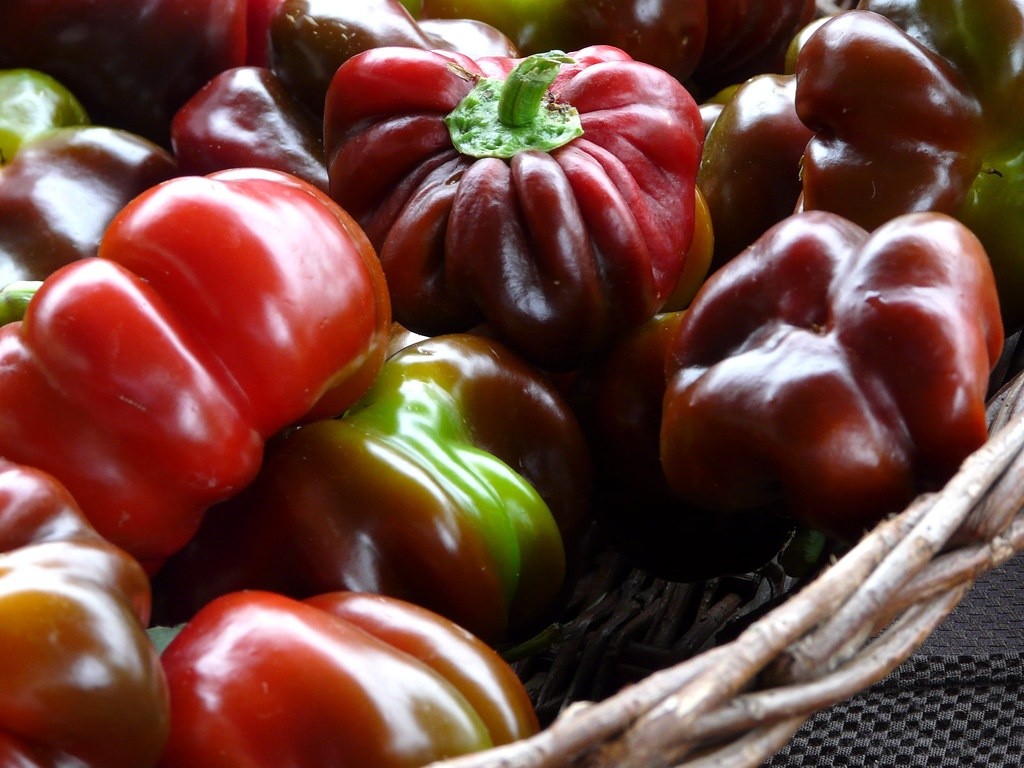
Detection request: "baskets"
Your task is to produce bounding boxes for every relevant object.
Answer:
[429,319,1024,768]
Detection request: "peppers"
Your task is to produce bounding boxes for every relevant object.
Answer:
[0,0,1024,768]
